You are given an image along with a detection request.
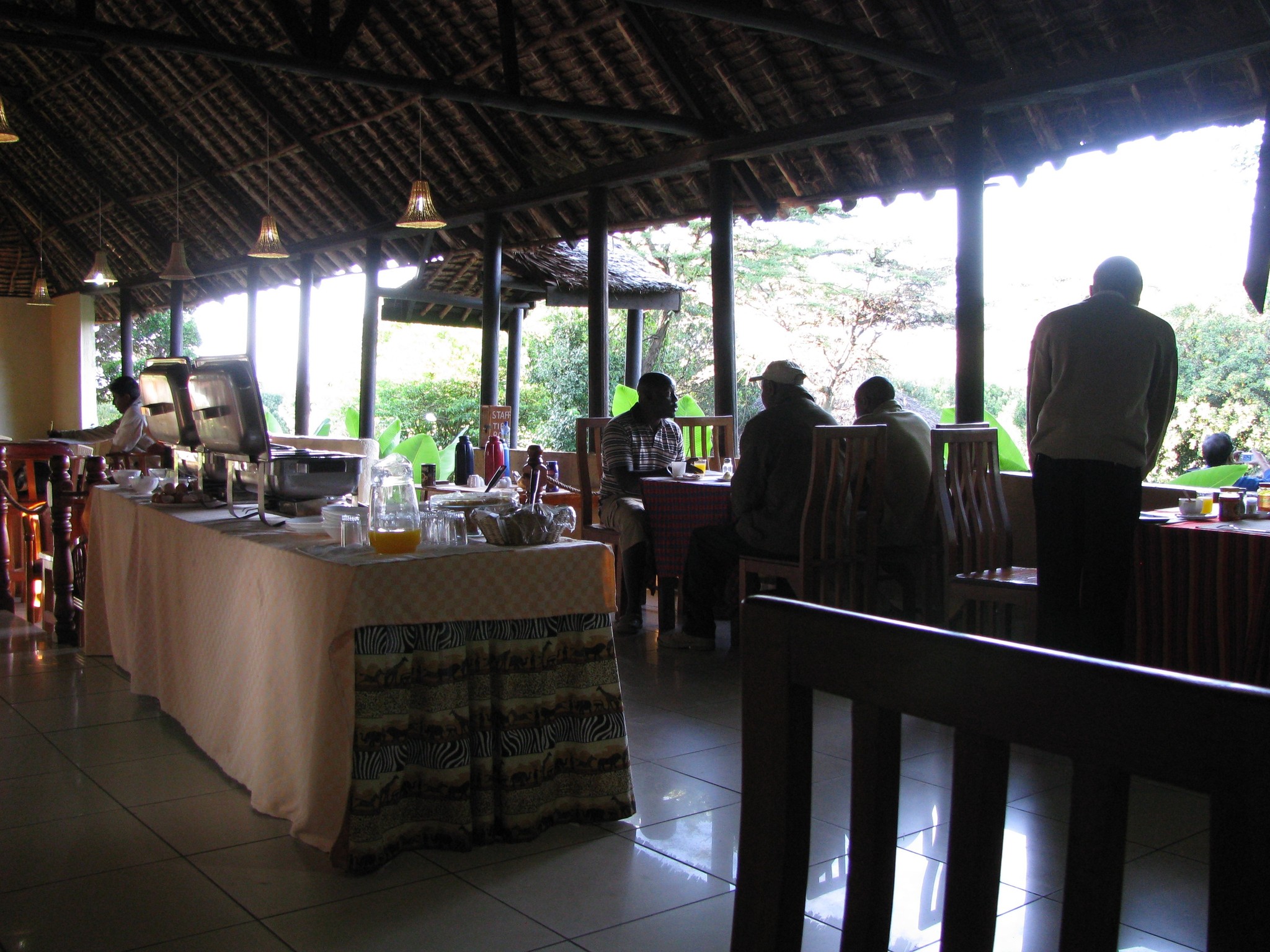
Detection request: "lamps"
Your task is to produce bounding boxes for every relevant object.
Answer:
[247,114,290,259]
[27,211,56,306]
[159,155,196,281]
[83,188,118,284]
[0,96,20,142]
[395,94,447,229]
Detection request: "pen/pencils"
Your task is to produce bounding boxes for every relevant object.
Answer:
[49,420,53,432]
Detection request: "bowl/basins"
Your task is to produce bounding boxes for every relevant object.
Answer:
[157,478,189,490]
[128,477,159,494]
[148,468,168,478]
[429,490,519,538]
[320,504,369,541]
[1179,498,1203,515]
[111,470,142,487]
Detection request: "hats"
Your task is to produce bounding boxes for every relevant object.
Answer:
[749,360,806,388]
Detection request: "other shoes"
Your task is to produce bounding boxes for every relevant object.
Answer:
[657,630,717,652]
[619,616,642,634]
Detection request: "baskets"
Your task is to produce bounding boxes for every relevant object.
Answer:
[471,508,572,544]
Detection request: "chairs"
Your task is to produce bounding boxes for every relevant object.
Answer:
[738,422,1038,646]
[0,442,162,631]
[732,596,1269,952]
[575,415,735,620]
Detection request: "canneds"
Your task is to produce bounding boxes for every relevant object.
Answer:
[1218,486,1240,522]
[421,463,436,487]
[1257,482,1270,512]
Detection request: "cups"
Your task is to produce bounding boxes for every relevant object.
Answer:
[1196,488,1213,514]
[467,474,484,487]
[422,463,436,486]
[502,476,512,487]
[694,459,707,477]
[339,514,363,550]
[417,510,467,548]
[667,461,686,479]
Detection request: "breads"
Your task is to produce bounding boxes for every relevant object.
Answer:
[152,482,199,503]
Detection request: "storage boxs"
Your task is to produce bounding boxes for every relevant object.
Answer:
[479,405,511,448]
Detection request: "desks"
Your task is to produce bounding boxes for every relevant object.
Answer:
[413,484,601,509]
[640,476,732,630]
[84,483,638,878]
[1133,506,1270,693]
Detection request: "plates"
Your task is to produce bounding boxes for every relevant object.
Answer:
[150,502,206,507]
[1244,511,1270,519]
[285,516,326,532]
[677,476,703,480]
[1177,513,1217,520]
[717,476,732,481]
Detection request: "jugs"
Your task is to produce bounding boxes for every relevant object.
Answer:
[367,453,422,554]
[500,439,510,478]
[455,435,474,485]
[485,436,505,485]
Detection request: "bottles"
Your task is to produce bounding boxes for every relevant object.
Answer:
[545,461,559,492]
[1219,482,1270,521]
[501,421,511,448]
[722,457,733,475]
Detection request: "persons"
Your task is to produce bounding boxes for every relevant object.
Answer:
[599,372,704,632]
[852,376,931,552]
[656,360,846,651]
[1201,433,1270,491]
[1027,257,1176,661]
[47,375,160,471]
